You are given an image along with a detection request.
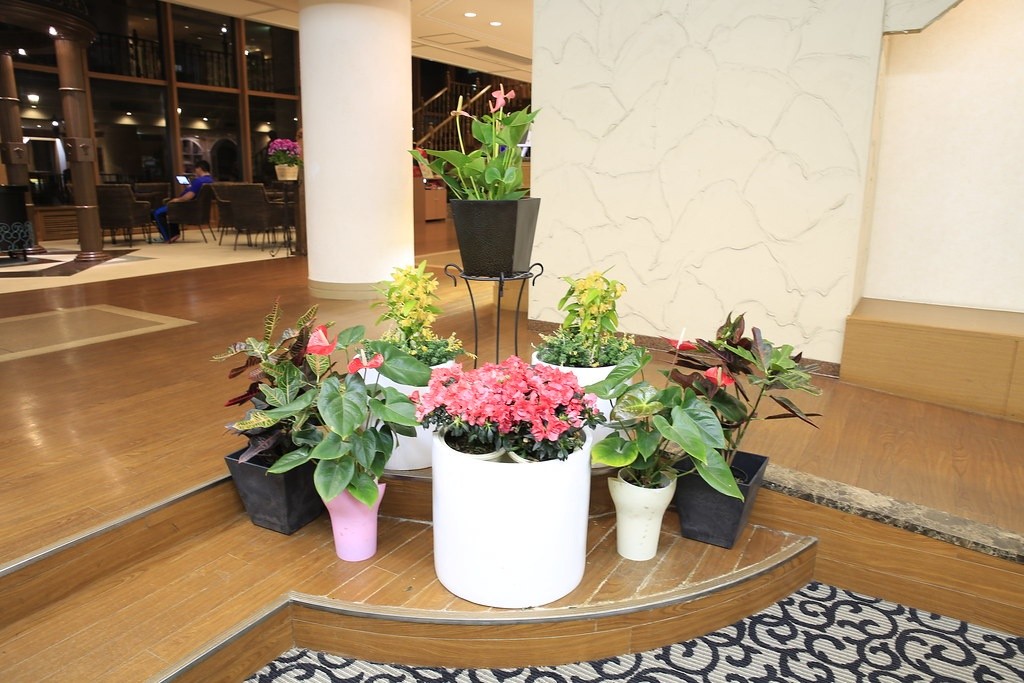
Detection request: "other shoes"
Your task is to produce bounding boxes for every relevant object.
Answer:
[146,235,165,243]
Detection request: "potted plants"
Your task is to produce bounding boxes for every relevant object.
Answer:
[658,310,823,550]
[207,295,340,534]
[581,348,746,563]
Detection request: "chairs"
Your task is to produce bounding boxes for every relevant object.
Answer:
[96,180,299,251]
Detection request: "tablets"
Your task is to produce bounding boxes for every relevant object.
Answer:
[176,175,190,185]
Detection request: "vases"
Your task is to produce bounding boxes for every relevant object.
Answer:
[355,354,458,469]
[449,197,541,276]
[320,483,387,561]
[275,164,298,181]
[431,430,593,608]
[531,349,632,460]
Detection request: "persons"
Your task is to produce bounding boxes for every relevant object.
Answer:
[152,160,212,243]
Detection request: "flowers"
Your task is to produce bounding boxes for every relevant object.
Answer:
[531,264,650,367]
[370,259,478,364]
[408,356,607,461]
[266,138,304,168]
[407,84,543,201]
[226,321,432,506]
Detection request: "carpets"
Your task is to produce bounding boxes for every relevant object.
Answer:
[233,580,1024,683]
